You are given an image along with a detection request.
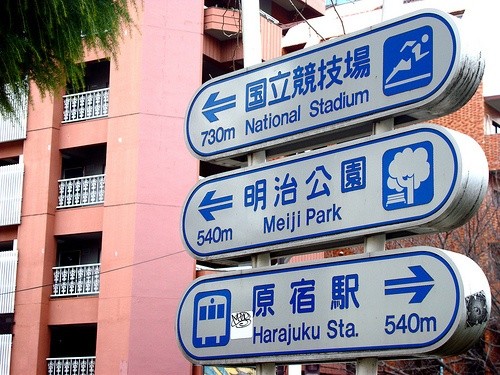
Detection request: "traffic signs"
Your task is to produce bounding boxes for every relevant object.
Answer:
[174,246,492,365]
[180,122,490,261]
[184,8,486,162]
